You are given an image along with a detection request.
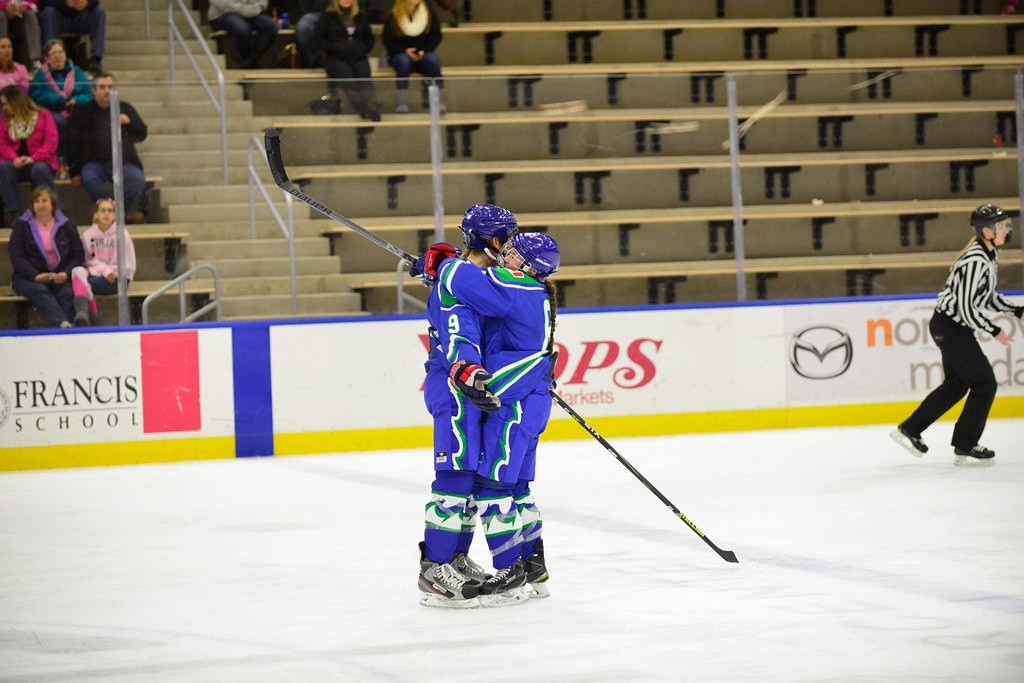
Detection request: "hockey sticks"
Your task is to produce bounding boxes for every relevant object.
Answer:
[264,127,419,264]
[550,389,753,564]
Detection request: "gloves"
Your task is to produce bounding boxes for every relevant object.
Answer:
[450,361,502,413]
[409,242,461,285]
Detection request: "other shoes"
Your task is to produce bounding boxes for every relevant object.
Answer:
[30,60,42,75]
[127,206,144,224]
[59,321,71,328]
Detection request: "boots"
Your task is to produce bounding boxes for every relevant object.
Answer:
[394,88,410,112]
[73,296,90,327]
[439,89,447,113]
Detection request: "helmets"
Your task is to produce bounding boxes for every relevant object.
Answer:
[461,204,519,250]
[971,205,1009,235]
[494,233,560,280]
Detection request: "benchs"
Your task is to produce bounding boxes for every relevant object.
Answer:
[218,1,1023,311]
[0,173,215,329]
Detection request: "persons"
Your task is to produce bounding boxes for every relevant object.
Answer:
[8,185,85,329]
[888,204,1024,467]
[0,85,60,226]
[0,0,42,73]
[65,72,147,221]
[206,0,448,113]
[409,203,560,608]
[71,197,137,324]
[37,0,106,74]
[28,38,95,133]
[0,36,29,96]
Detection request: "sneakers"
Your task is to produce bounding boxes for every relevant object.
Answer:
[954,439,995,467]
[521,539,550,599]
[451,553,491,583]
[890,422,929,460]
[417,541,479,610]
[477,542,533,607]
